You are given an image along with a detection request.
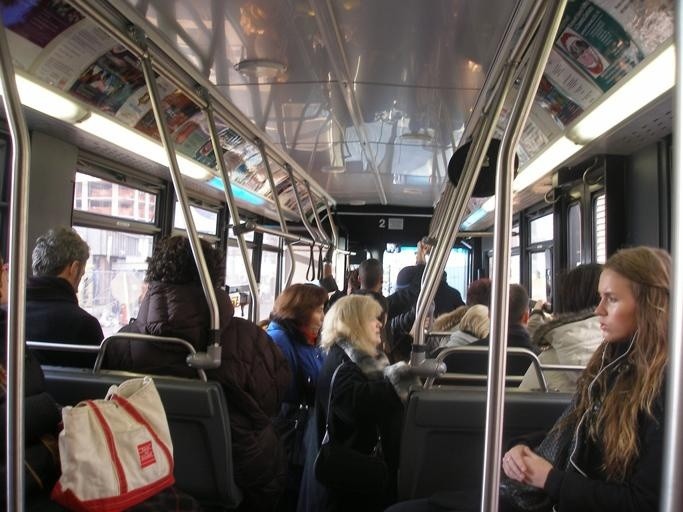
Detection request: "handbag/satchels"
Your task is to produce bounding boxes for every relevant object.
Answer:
[50,377,176,511]
[498,395,578,509]
[312,360,386,497]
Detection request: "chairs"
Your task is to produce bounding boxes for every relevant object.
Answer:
[396,347,575,500]
[27,332,243,505]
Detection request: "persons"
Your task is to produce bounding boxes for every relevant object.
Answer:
[527,299,547,336]
[354,259,387,291]
[384,265,463,316]
[386,247,674,512]
[421,284,542,388]
[266,283,329,511]
[319,243,360,312]
[430,277,492,331]
[119,236,279,511]
[444,305,489,346]
[318,294,404,511]
[25,227,104,368]
[518,263,607,395]
